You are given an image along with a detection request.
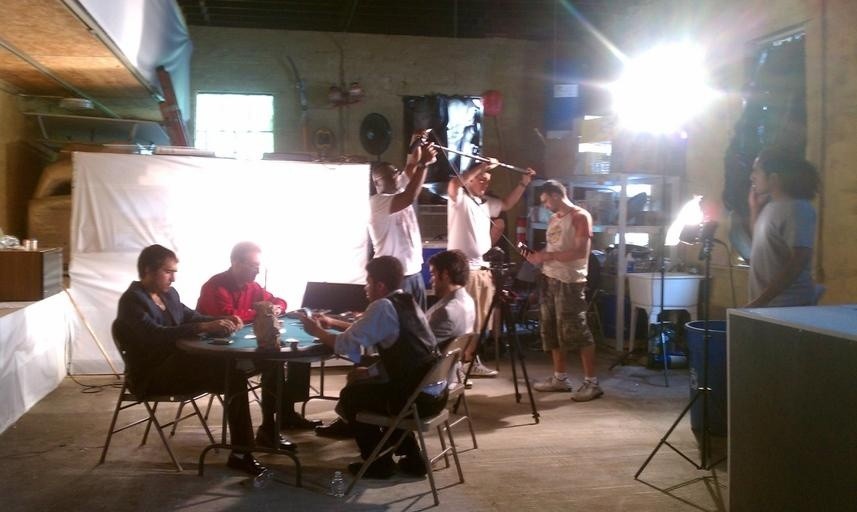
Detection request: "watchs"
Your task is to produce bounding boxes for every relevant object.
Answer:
[416,161,428,169]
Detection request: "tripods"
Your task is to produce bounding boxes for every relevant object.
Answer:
[634,243,726,480]
[610,177,687,386]
[453,248,540,424]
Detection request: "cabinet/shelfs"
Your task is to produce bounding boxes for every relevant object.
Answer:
[526,172,680,354]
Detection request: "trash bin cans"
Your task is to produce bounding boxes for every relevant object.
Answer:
[684,320,728,437]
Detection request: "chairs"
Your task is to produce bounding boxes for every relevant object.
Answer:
[298,281,373,421]
[97,316,222,471]
[341,331,478,504]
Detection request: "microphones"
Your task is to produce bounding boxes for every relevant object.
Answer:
[408,128,431,154]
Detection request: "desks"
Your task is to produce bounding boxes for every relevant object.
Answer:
[627,272,712,357]
[175,309,362,487]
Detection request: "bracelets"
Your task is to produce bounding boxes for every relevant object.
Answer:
[517,181,529,189]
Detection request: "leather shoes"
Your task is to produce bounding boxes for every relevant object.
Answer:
[227,412,427,478]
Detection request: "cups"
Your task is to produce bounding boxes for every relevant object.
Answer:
[251,301,276,349]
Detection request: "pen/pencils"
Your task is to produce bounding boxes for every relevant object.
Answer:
[323,309,332,314]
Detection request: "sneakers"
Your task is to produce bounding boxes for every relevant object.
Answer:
[462,354,499,377]
[533,376,572,392]
[571,381,603,402]
[457,368,473,389]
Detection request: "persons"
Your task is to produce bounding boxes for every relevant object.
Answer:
[196,241,325,451]
[366,127,436,313]
[526,250,602,352]
[477,195,509,366]
[117,243,268,474]
[517,180,602,401]
[295,256,437,481]
[744,144,823,309]
[445,157,539,388]
[314,249,478,442]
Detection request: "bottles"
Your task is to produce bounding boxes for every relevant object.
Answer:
[254,468,272,487]
[330,471,345,499]
[22,239,38,252]
